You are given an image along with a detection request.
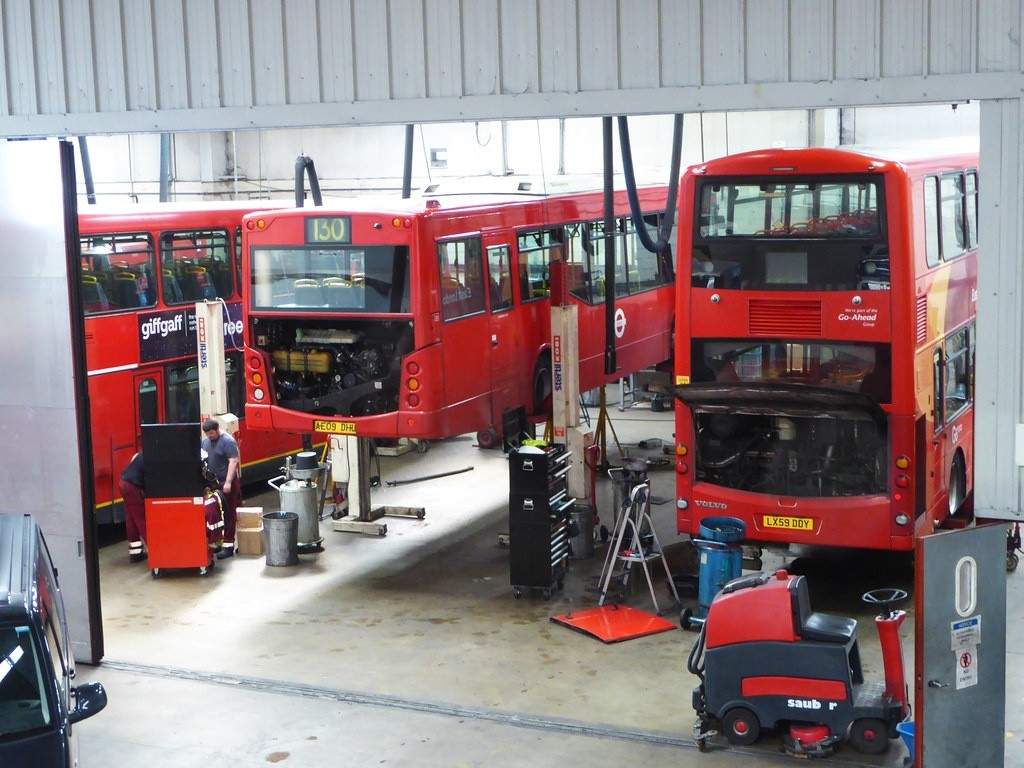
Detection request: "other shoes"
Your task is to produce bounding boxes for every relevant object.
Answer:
[130,551,147,563]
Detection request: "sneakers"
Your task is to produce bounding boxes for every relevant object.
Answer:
[213,544,222,552]
[216,546,234,559]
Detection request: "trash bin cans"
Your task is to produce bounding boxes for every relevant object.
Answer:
[568,503,594,558]
[261,511,300,567]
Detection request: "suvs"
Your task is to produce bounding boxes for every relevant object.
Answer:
[0,510,108,768]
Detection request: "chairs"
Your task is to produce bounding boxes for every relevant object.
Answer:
[294,274,503,320]
[82,255,241,312]
[789,574,858,643]
[752,207,876,236]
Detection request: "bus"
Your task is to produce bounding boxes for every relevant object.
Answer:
[75,196,452,528]
[241,181,718,442]
[670,137,979,591]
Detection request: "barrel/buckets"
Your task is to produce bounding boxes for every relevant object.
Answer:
[569,504,593,561]
[651,393,663,411]
[262,513,300,566]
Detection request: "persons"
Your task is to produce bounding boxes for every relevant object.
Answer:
[118,420,241,563]
